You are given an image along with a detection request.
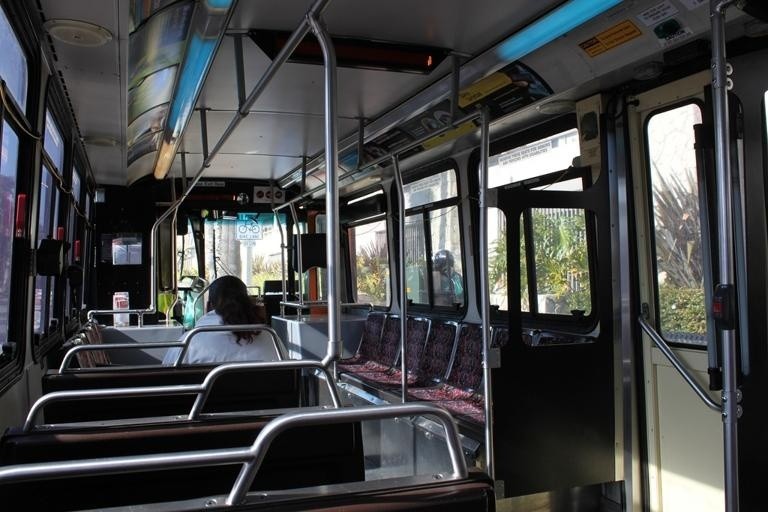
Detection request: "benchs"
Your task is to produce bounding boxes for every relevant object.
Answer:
[1,324,496,512]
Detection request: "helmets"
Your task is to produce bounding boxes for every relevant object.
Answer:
[434,250,454,270]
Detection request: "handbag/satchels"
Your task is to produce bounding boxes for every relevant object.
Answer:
[183,288,204,331]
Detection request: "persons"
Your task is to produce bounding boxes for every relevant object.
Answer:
[432,250,464,307]
[160,275,290,367]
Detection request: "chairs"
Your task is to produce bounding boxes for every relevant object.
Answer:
[314,309,575,456]
